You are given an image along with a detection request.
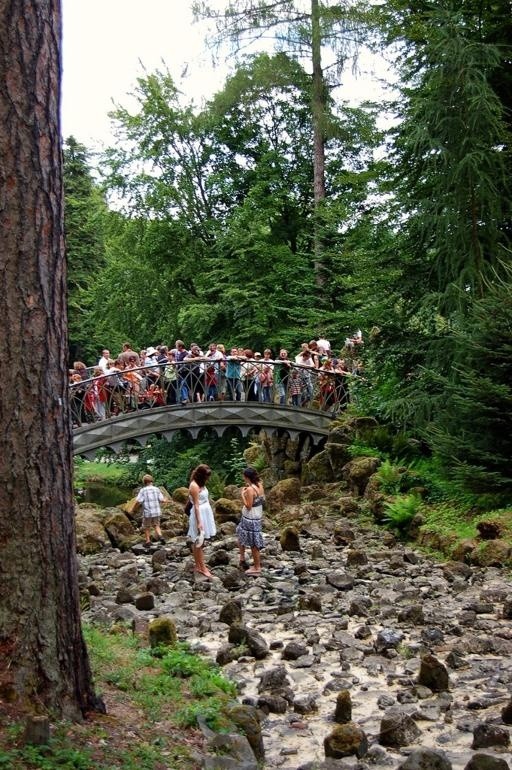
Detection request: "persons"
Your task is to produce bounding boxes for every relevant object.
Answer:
[235,466,265,574]
[67,328,365,431]
[129,473,167,549]
[185,463,217,580]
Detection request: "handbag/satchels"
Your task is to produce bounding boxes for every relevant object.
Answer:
[185,501,193,516]
[252,495,266,507]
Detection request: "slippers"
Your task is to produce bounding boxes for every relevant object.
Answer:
[195,531,206,549]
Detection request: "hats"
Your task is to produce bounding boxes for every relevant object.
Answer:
[145,347,157,357]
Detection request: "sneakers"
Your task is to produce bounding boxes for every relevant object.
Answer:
[143,537,165,547]
